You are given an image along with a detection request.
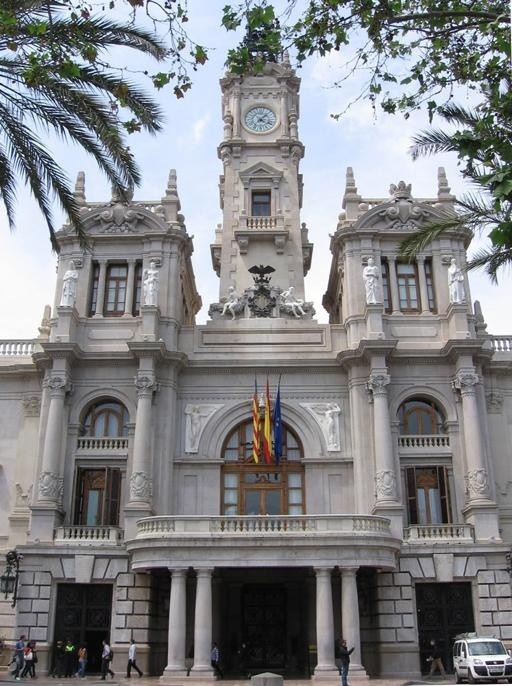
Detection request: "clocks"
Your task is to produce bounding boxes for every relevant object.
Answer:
[240,101,280,134]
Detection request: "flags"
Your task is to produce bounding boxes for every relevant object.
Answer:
[273,384,282,464]
[251,393,262,464]
[263,383,272,464]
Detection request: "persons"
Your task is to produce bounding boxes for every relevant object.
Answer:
[185,406,209,436]
[340,640,355,686]
[280,287,306,318]
[211,640,224,679]
[448,259,465,304]
[124,640,143,678]
[75,640,88,678]
[429,639,446,680]
[64,641,75,678]
[52,640,65,678]
[11,635,38,680]
[363,258,380,305]
[221,286,238,320]
[60,261,78,308]
[316,404,341,446]
[143,262,160,307]
[101,640,115,680]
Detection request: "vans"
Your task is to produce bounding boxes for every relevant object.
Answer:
[452,637,512,684]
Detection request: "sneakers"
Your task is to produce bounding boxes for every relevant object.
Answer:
[47,673,87,681]
[11,674,37,682]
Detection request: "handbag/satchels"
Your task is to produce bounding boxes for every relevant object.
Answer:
[109,651,114,660]
[24,652,33,661]
[34,652,38,663]
